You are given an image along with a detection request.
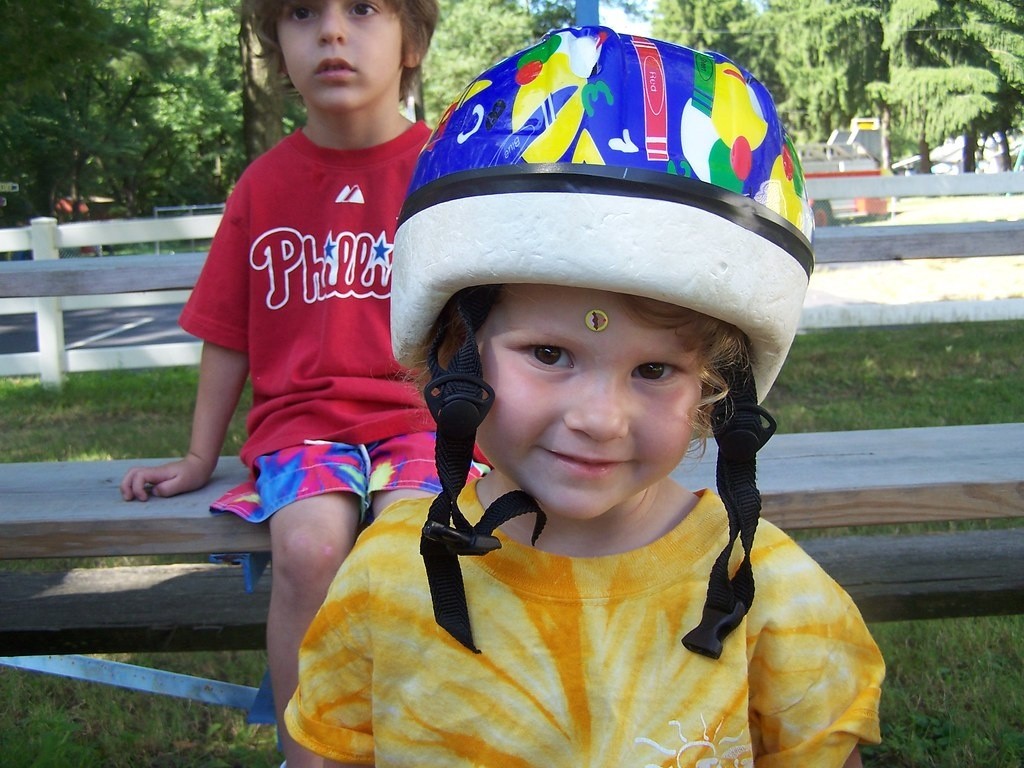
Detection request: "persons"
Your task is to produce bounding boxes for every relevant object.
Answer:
[120,0,494,768]
[285,24,886,768]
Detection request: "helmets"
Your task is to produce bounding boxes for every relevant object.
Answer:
[390,25,815,406]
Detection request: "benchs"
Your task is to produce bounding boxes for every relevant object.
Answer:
[0,222,1024,657]
